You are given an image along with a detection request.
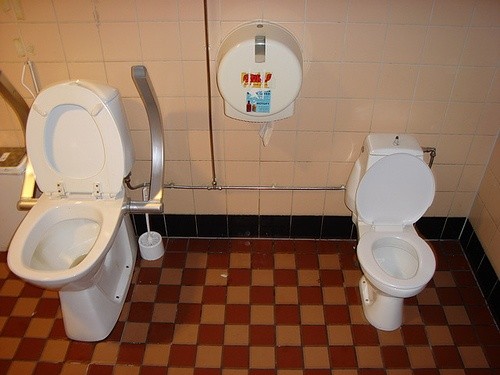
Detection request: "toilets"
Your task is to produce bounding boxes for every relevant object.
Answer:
[6,78,138,343]
[343,132,436,331]
